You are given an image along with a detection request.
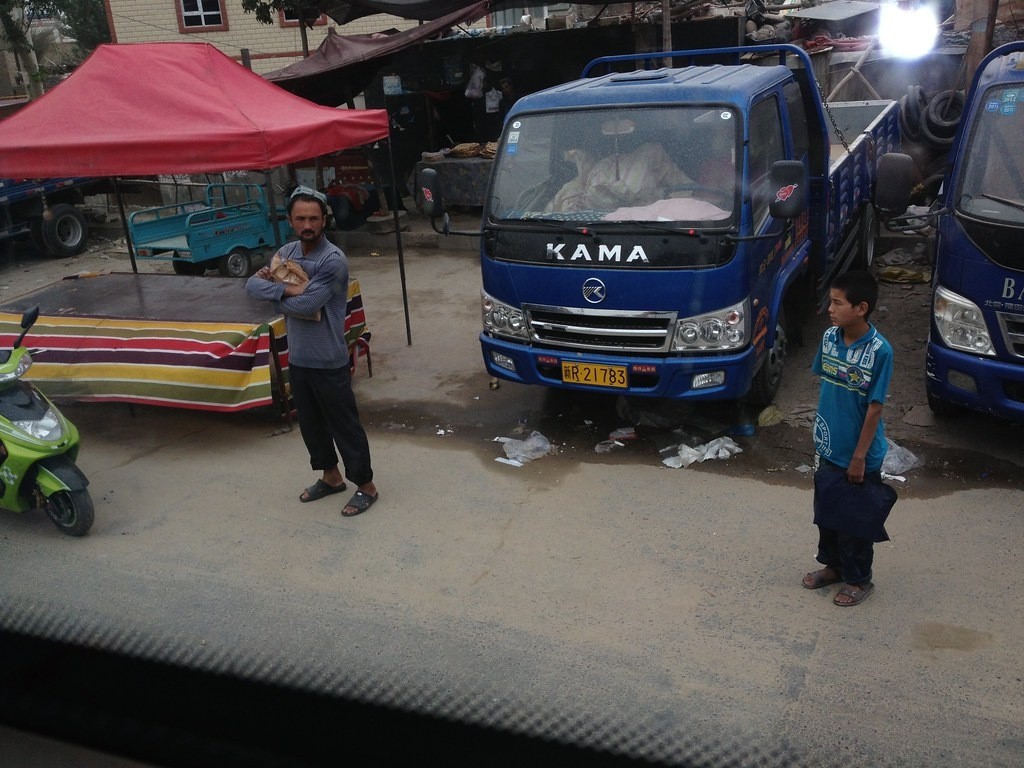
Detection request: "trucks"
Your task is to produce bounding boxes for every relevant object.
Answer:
[416,42,886,408]
[0,176,108,258]
[877,41,1023,419]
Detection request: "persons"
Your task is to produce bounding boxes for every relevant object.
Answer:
[801,267,892,606]
[246,185,380,515]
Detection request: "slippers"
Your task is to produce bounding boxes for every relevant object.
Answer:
[300,479,346,502]
[341,491,379,516]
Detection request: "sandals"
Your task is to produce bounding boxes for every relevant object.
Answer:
[834,583,874,606]
[802,568,839,589]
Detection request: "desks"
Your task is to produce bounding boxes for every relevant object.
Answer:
[7,271,374,431]
[407,155,494,214]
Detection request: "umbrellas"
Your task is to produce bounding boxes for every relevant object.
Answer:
[261,0,492,111]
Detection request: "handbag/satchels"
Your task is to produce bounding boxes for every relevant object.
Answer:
[466,67,484,98]
[813,461,898,543]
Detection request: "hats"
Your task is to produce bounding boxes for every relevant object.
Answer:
[291,185,328,210]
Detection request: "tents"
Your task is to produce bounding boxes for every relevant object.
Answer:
[0,44,412,349]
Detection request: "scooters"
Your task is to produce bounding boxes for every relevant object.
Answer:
[0,303,96,538]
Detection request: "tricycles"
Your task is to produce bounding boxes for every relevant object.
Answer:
[126,183,342,278]
